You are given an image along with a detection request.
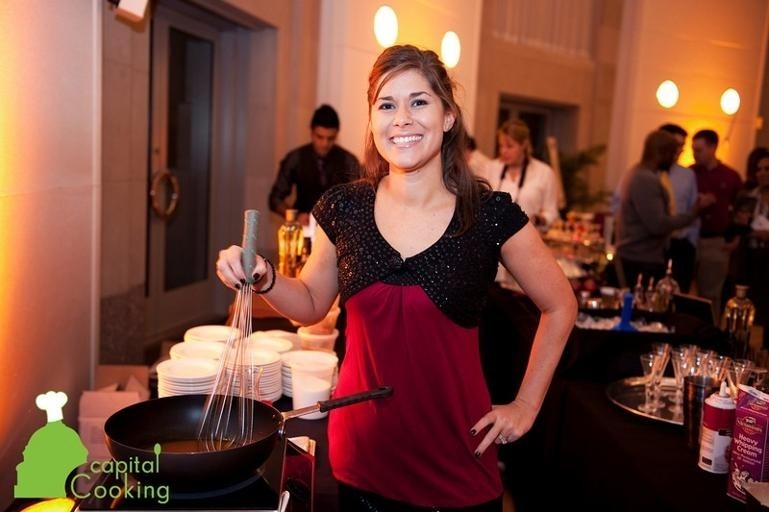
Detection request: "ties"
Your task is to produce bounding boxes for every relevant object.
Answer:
[317,158,327,186]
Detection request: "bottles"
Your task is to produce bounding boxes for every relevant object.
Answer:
[698,381,735,475]
[722,285,755,335]
[635,269,679,310]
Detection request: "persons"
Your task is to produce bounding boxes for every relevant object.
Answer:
[477,117,560,283]
[609,121,769,324]
[267,102,361,253]
[216,43,580,511]
[462,130,492,177]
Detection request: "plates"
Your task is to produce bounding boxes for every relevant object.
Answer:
[156,324,338,405]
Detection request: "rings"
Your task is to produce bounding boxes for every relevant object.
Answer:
[498,432,509,445]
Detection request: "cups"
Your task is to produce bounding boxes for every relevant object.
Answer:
[287,348,339,421]
[683,376,714,432]
[600,286,620,308]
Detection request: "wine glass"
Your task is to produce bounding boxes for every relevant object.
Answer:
[638,343,769,413]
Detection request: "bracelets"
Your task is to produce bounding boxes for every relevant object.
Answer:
[251,251,276,294]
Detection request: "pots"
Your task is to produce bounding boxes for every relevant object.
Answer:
[103,385,393,494]
